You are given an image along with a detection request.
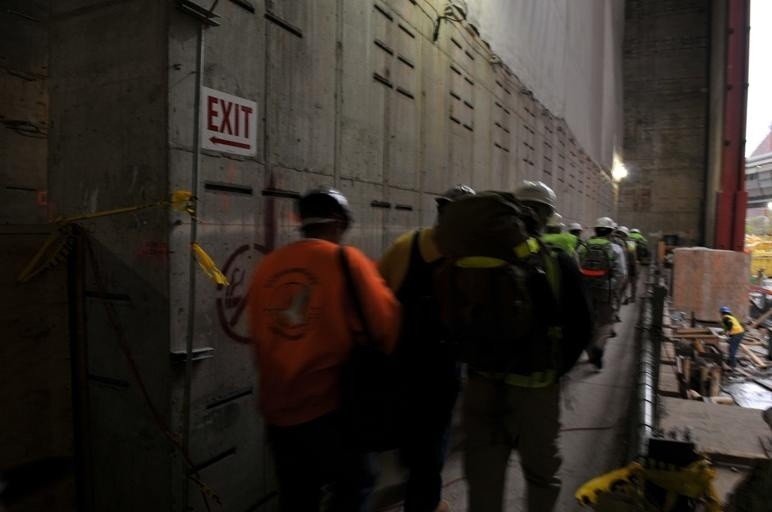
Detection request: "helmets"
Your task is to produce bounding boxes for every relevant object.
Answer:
[293,185,355,227]
[613,222,618,229]
[567,222,584,232]
[630,228,641,234]
[512,179,559,214]
[433,184,478,209]
[593,215,616,231]
[616,226,630,237]
[545,212,565,229]
[719,306,731,313]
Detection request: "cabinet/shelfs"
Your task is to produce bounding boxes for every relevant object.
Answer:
[657,241,679,265]
[42,1,617,512]
[0,0,75,512]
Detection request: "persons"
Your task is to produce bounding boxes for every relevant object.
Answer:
[718,303,745,367]
[246,183,402,511]
[462,180,595,512]
[546,212,649,370]
[375,184,478,512]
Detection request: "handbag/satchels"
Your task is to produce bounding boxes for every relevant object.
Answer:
[336,344,413,452]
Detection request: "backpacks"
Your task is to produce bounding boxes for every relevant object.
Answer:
[581,242,614,290]
[434,188,550,370]
